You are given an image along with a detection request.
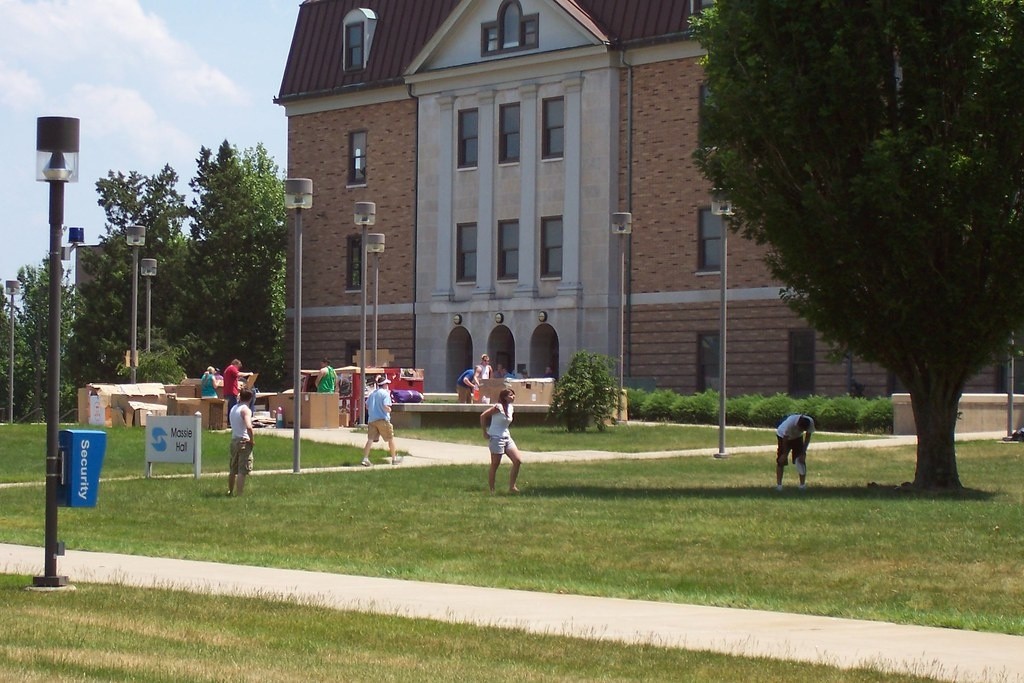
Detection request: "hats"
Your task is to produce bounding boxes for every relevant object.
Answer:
[377,379,392,386]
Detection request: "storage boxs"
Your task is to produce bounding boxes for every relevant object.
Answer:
[338,412,350,426]
[478,378,555,404]
[253,411,270,418]
[78,374,258,430]
[268,393,339,428]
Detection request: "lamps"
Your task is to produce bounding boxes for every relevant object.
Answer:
[453,314,462,325]
[539,311,547,322]
[495,313,504,323]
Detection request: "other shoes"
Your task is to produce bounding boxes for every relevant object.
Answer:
[362,460,373,466]
[392,458,402,465]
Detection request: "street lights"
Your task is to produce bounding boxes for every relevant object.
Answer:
[283,177,314,473]
[141,257,159,359]
[6,279,20,424]
[365,232,387,368]
[126,224,147,388]
[28,115,81,586]
[709,185,738,459]
[352,200,378,427]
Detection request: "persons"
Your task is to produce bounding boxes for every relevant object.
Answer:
[228,388,257,496]
[223,359,254,424]
[456,367,482,404]
[475,355,494,379]
[494,362,513,377]
[315,359,339,392]
[544,366,557,378]
[362,377,403,466]
[201,366,223,399]
[775,415,814,491]
[480,389,522,492]
